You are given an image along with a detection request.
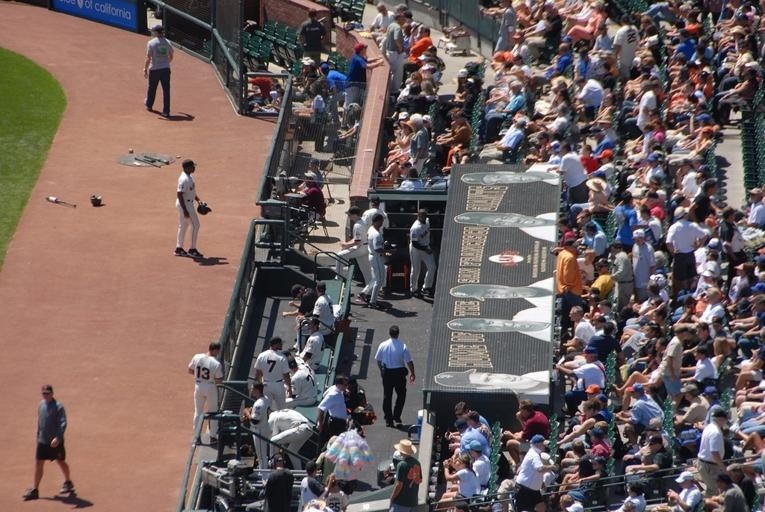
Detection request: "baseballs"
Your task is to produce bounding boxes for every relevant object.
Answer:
[129,149,133,153]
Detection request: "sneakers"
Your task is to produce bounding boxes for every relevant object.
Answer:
[172,247,189,257]
[208,436,219,446]
[144,97,153,111]
[158,111,170,117]
[20,487,39,500]
[354,282,433,309]
[57,478,77,495]
[186,248,204,259]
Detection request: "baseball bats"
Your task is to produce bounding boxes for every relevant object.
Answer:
[47,196,76,207]
[135,157,161,167]
[144,156,169,164]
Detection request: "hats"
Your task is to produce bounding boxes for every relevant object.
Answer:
[278,155,322,186]
[481,0,552,163]
[448,398,552,512]
[342,192,427,223]
[90,191,103,206]
[376,2,480,180]
[253,280,375,512]
[553,0,765,512]
[151,23,162,31]
[197,203,211,215]
[394,438,417,455]
[299,7,368,76]
[269,336,286,346]
[39,384,53,395]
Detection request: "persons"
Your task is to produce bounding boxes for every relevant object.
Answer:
[185,338,226,448]
[171,158,208,263]
[20,384,75,501]
[140,25,173,119]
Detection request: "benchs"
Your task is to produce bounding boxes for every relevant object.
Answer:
[195,0,765,512]
